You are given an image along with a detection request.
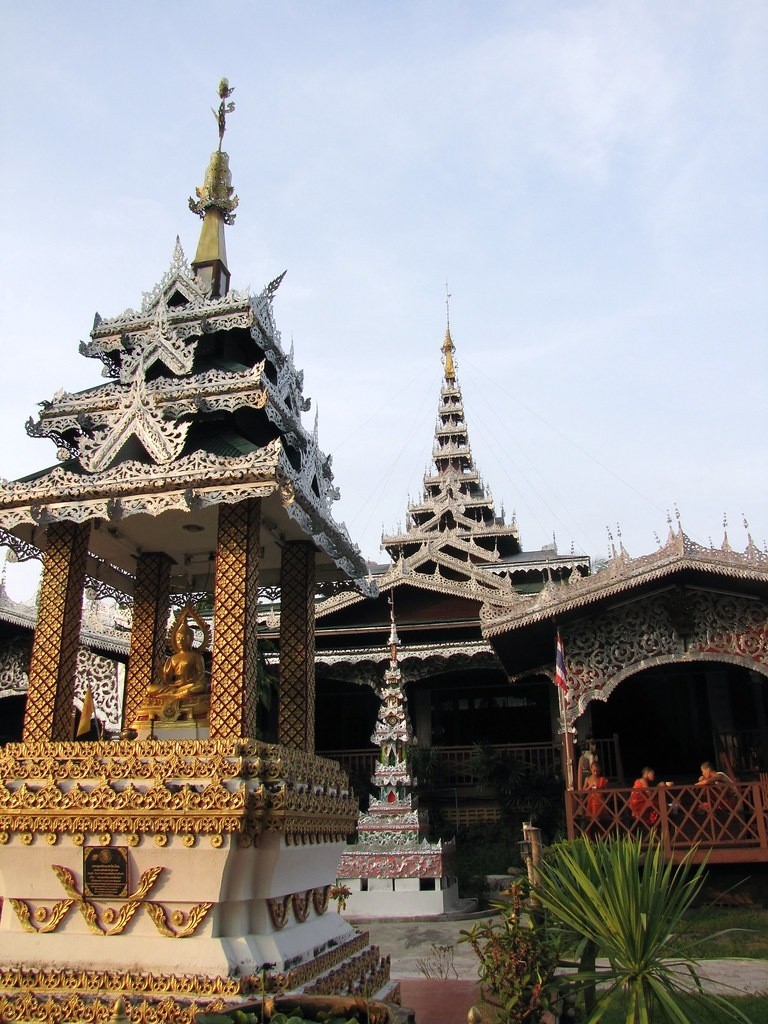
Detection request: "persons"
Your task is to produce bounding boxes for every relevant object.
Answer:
[577,735,739,826]
[146,624,207,698]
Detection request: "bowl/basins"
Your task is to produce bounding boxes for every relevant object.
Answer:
[666,781,674,787]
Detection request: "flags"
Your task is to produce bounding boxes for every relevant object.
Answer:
[553,634,570,696]
[76,685,94,737]
